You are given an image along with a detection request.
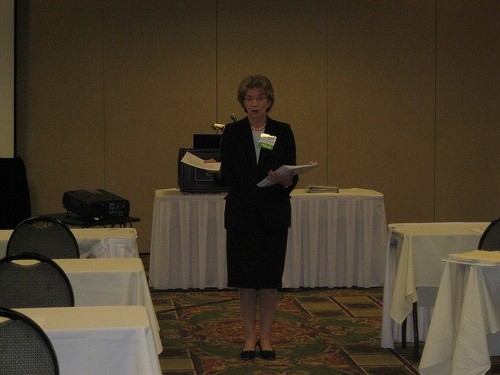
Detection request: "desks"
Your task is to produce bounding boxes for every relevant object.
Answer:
[378,221,492,360]
[0,226,141,258]
[0,256,164,355]
[149,184,388,294]
[0,308,162,375]
[416,248,500,375]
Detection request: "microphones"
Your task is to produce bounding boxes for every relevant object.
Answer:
[231,112,237,121]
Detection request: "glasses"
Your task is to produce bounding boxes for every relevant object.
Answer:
[244,95,268,101]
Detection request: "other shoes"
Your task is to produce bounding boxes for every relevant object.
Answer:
[257,337,277,361]
[241,337,259,358]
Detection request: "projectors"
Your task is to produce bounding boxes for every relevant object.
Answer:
[62,188,130,220]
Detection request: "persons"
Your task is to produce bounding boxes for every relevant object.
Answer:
[218,74,300,361]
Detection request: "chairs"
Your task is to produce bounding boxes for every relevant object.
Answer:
[0,306,61,375]
[478,217,500,250]
[0,251,75,307]
[5,216,81,260]
